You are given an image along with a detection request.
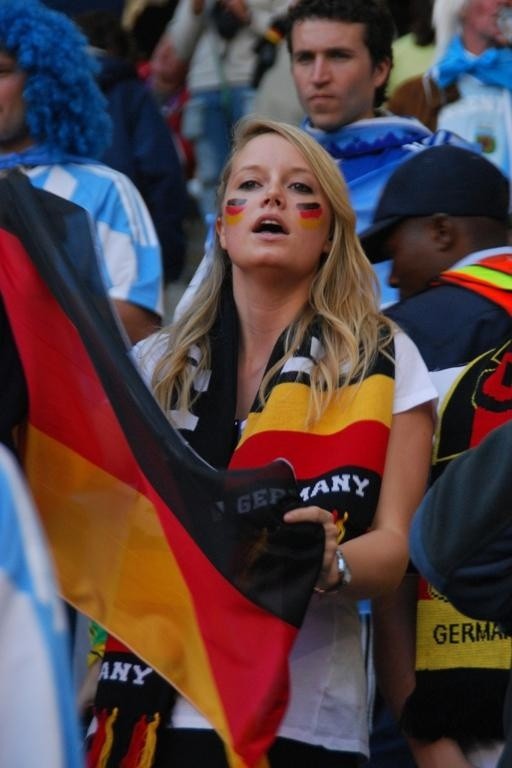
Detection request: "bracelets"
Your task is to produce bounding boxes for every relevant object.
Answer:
[335,550,352,585]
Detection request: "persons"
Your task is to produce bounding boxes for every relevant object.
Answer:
[129,118,439,768]
[356,146,511,768]
[1,1,512,346]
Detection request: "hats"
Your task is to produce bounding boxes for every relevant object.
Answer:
[358,145,511,263]
